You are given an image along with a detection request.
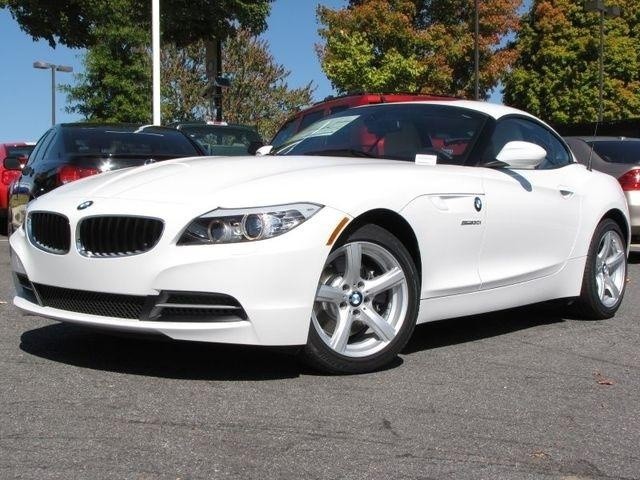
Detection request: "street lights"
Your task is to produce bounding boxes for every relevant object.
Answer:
[34,60,72,126]
